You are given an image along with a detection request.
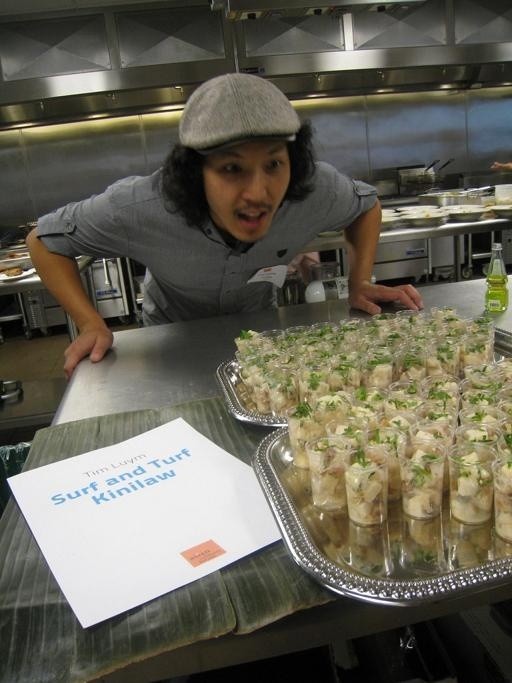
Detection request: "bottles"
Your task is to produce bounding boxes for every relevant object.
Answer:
[484,240,511,312]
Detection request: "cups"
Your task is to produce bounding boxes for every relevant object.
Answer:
[234,305,512,542]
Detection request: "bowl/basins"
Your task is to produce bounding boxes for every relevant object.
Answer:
[379,202,512,233]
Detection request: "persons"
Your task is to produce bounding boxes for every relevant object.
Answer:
[26,73,425,380]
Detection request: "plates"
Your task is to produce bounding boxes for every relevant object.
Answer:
[0,250,36,281]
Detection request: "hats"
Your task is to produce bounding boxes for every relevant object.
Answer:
[178,71,301,155]
